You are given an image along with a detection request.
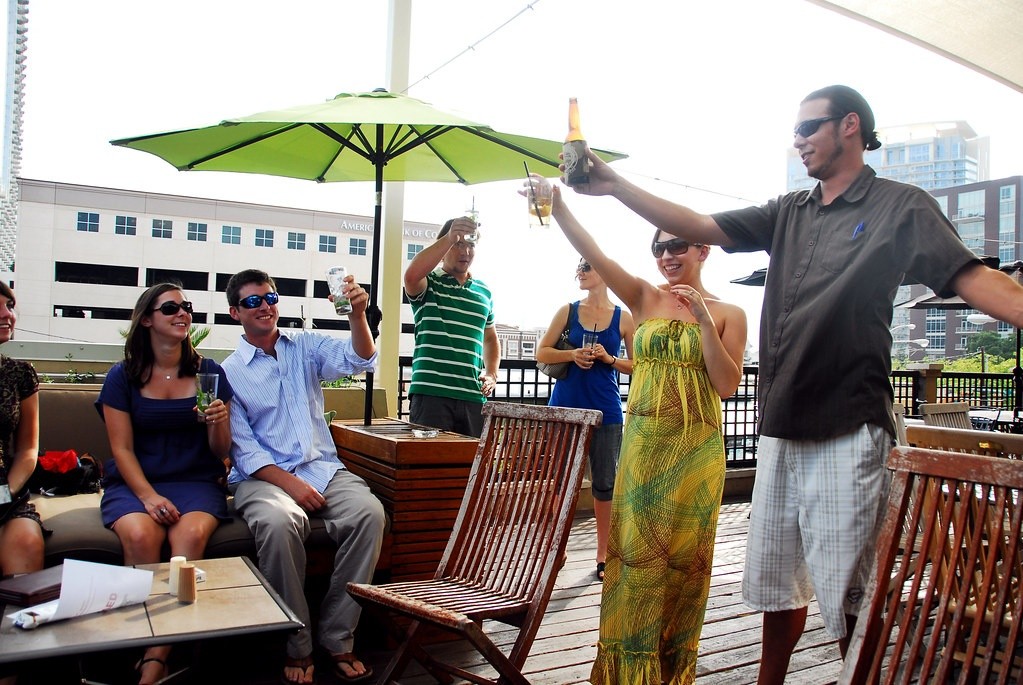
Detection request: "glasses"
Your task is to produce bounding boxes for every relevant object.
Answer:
[577,263,591,273]
[652,238,710,258]
[152,301,193,315]
[233,292,279,309]
[798,115,846,138]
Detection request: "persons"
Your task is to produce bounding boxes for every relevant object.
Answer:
[220,269,386,685]
[404,216,501,439]
[0,281,53,577]
[94,283,235,685]
[518,172,747,685]
[536,257,636,582]
[559,85,1023,685]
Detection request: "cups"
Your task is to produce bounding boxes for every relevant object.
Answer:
[325,266,353,316]
[526,177,553,229]
[196,373,219,420]
[583,333,598,361]
[464,210,480,242]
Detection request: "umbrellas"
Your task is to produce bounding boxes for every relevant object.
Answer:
[109,87,628,426]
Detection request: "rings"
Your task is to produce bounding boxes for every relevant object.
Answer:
[688,290,694,295]
[160,509,168,515]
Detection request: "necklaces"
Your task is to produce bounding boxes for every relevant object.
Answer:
[156,365,178,380]
[676,301,683,310]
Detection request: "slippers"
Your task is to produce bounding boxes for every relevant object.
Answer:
[283,652,371,685]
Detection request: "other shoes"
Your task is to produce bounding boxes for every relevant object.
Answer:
[597,562,605,581]
[133,656,173,685]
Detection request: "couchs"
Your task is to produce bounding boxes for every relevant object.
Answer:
[27,389,391,657]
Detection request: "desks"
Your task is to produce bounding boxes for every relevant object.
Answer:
[912,483,1023,627]
[966,409,1023,431]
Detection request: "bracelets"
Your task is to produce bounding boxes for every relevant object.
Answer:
[610,355,616,366]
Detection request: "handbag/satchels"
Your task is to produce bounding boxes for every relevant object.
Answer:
[26,448,103,496]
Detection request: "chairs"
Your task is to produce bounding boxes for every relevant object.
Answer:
[906,424,1023,685]
[836,446,1023,685]
[919,400,978,484]
[891,402,988,618]
[345,399,604,685]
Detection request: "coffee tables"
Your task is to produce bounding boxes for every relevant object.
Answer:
[0,555,306,685]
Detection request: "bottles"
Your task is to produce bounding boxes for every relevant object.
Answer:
[563,97,590,185]
